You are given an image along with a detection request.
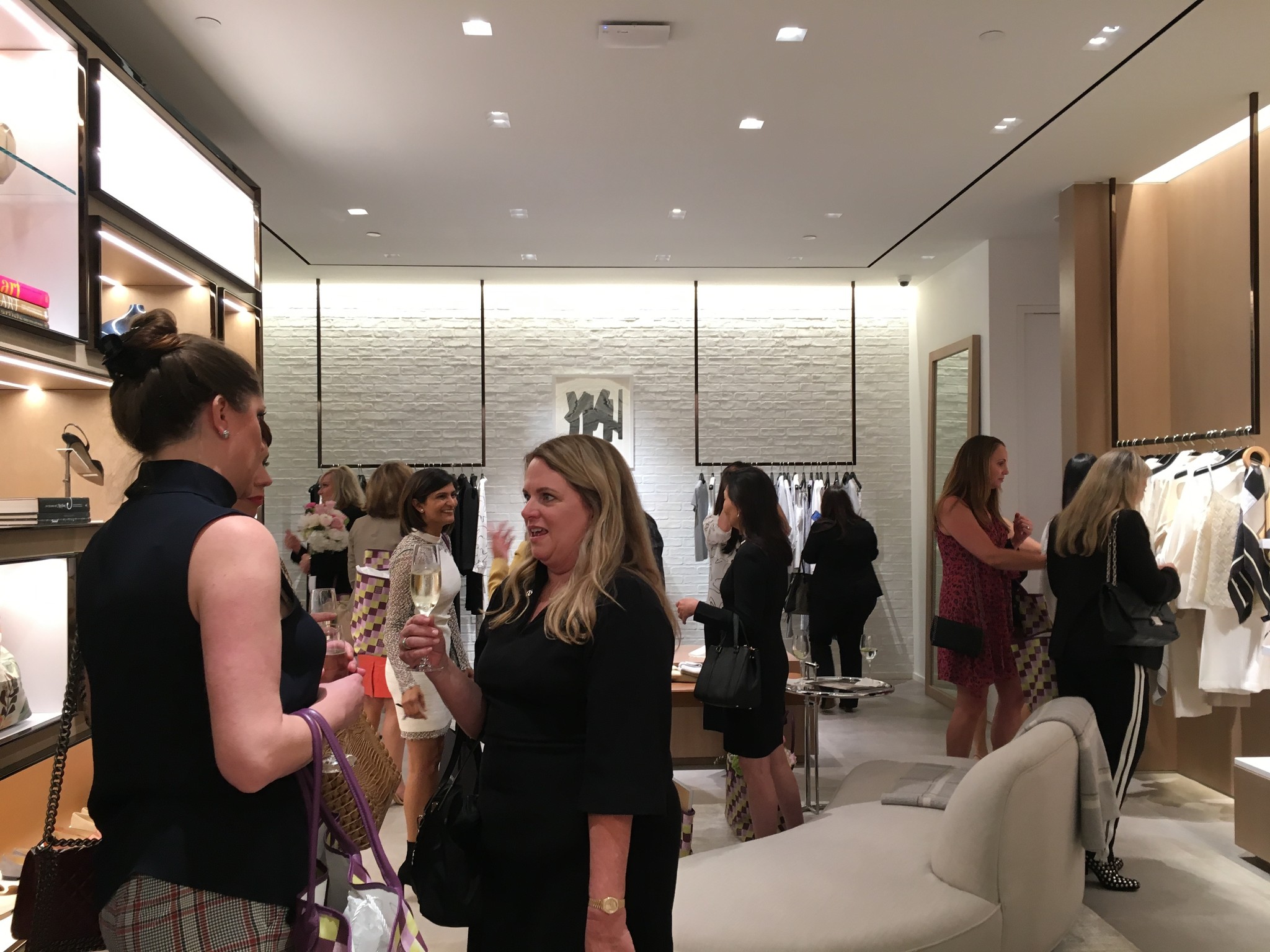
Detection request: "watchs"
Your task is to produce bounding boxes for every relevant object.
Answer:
[588,897,625,913]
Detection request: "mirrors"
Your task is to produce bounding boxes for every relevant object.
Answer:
[924,336,978,711]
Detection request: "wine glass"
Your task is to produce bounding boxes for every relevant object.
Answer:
[860,635,878,683]
[792,635,811,685]
[405,545,444,672]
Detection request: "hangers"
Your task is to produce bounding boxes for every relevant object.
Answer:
[1116,426,1270,481]
[319,463,484,492]
[699,462,862,491]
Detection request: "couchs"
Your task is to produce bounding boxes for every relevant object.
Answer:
[673,700,1097,952]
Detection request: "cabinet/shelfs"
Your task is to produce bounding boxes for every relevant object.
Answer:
[670,645,818,760]
[1232,756,1270,863]
[1,1,266,952]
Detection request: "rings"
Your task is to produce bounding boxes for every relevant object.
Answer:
[403,637,411,649]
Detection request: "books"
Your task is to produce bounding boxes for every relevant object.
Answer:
[0,274,50,328]
[0,497,91,525]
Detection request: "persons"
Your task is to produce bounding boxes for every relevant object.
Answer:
[703,461,792,608]
[74,308,366,952]
[1047,448,1182,891]
[801,486,884,710]
[485,519,533,601]
[675,466,804,839]
[346,461,414,773]
[284,465,367,647]
[383,468,474,898]
[397,435,684,952]
[933,436,1047,758]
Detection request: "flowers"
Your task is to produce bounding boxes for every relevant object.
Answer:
[295,499,349,555]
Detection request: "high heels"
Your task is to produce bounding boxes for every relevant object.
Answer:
[1114,857,1124,870]
[1084,854,1140,891]
[398,841,416,897]
[62,424,104,483]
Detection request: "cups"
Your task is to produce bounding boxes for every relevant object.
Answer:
[320,624,348,683]
[308,588,338,630]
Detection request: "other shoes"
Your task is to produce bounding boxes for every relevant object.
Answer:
[839,702,853,713]
[820,698,836,709]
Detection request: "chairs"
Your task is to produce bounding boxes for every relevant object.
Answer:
[785,676,894,815]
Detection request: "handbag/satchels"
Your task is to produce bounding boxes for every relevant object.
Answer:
[12,838,135,952]
[350,549,393,658]
[289,708,428,952]
[785,555,814,615]
[678,807,696,858]
[931,615,982,657]
[724,753,787,841]
[322,711,402,850]
[1101,508,1179,646]
[1010,593,1059,706]
[399,723,486,927]
[694,613,761,709]
